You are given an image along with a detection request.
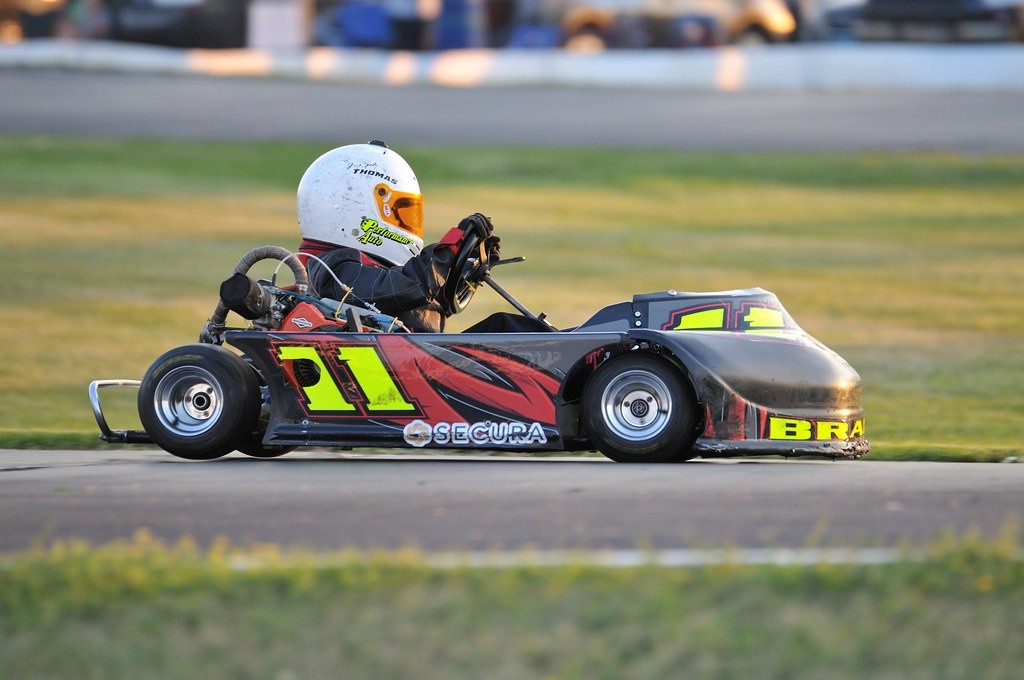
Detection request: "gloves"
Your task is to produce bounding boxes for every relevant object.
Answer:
[470,236,500,264]
[458,212,493,245]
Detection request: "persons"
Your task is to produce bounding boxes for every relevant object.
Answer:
[297,142,579,332]
[0,0,797,51]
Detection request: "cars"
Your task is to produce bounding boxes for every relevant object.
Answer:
[0,0,1023,54]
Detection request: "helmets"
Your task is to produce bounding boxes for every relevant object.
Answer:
[297,144,424,267]
